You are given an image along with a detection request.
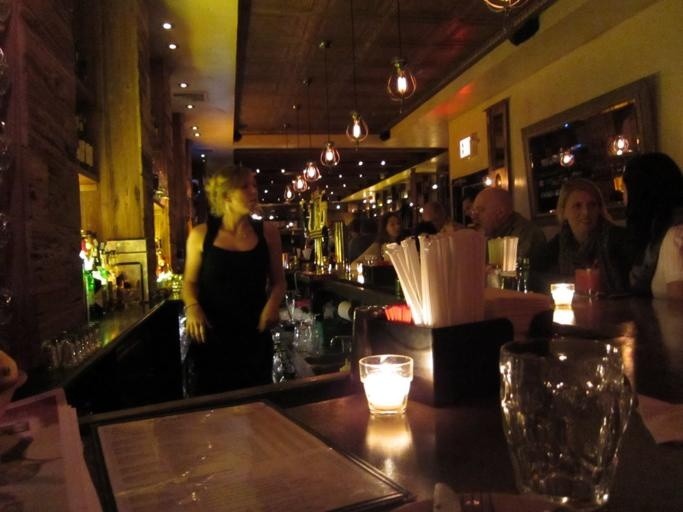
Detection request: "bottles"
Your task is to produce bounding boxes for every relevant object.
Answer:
[82,230,125,323]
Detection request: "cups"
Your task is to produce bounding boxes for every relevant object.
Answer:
[358,353,413,414]
[494,335,636,508]
[552,306,576,327]
[549,282,575,310]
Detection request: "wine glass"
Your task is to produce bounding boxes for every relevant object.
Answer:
[286,291,296,320]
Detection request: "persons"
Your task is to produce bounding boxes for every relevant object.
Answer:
[179,164,288,397]
[621,152,683,298]
[460,186,547,272]
[351,202,451,269]
[537,178,630,275]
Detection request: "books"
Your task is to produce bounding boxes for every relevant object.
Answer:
[1,386,400,510]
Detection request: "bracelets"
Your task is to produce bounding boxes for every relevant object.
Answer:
[182,300,204,310]
